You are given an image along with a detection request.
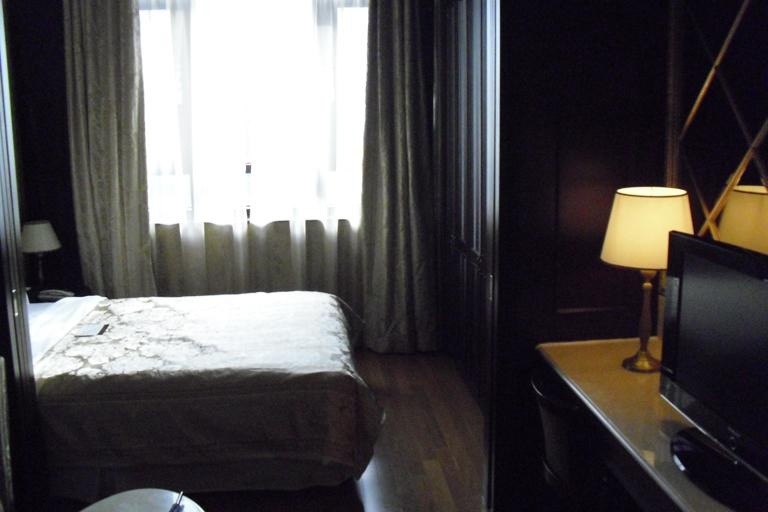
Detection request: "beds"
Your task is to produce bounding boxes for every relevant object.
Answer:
[28,291,386,501]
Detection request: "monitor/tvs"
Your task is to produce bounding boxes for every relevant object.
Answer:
[660,230,768,512]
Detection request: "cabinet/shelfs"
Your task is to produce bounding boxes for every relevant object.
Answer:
[1,12,42,511]
[430,0,664,511]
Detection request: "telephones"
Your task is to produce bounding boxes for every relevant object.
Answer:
[38,290,75,301]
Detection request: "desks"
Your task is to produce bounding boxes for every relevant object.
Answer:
[535,334,730,512]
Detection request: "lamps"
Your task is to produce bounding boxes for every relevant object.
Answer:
[601,187,695,373]
[719,185,768,257]
[22,219,62,289]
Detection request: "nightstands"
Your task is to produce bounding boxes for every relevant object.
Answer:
[26,286,90,300]
